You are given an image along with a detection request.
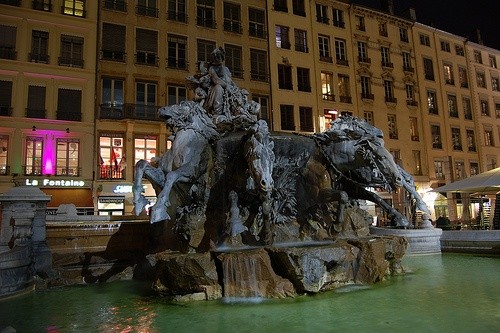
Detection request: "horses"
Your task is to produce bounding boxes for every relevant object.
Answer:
[131,102,410,237]
